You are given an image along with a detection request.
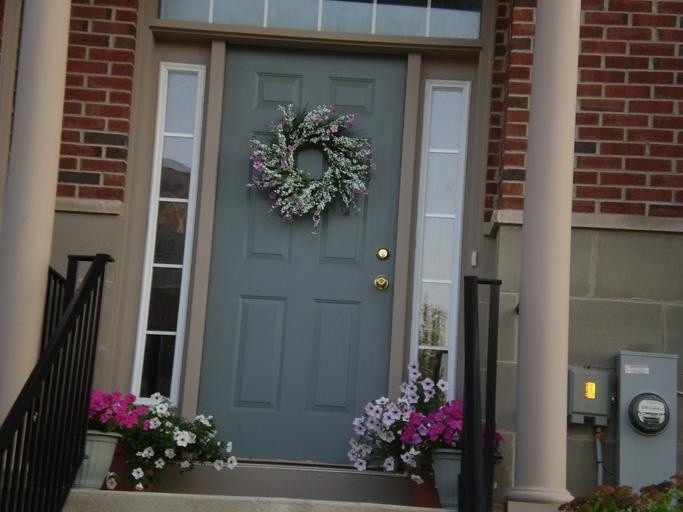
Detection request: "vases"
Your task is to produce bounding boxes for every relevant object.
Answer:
[403,450,463,505]
[69,430,128,491]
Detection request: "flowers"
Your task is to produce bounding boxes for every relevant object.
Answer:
[346,361,504,482]
[249,99,375,232]
[88,386,239,493]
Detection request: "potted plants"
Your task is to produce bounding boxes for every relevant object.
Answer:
[414,298,444,408]
